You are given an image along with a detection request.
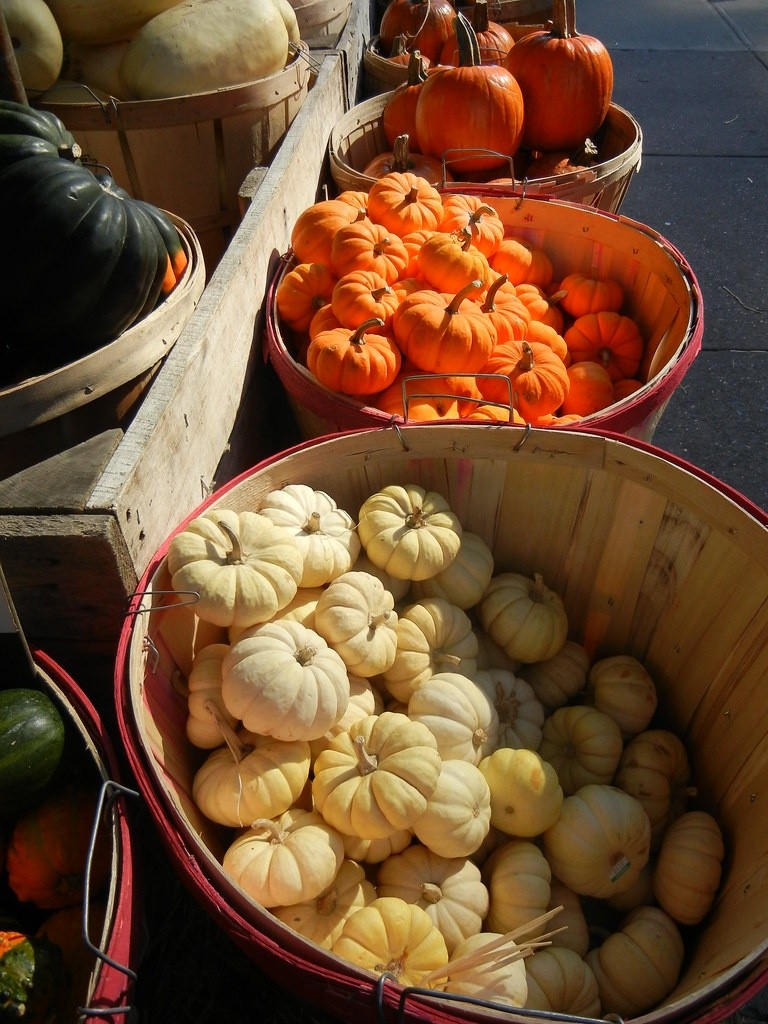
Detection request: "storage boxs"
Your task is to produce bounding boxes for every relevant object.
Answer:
[362,20,554,101]
[294,0,353,50]
[327,90,643,218]
[0,207,206,480]
[31,36,311,290]
[0,645,137,1023]
[110,416,768,1024]
[259,187,705,443]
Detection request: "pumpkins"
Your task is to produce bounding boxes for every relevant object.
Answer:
[0,0,724,1024]
